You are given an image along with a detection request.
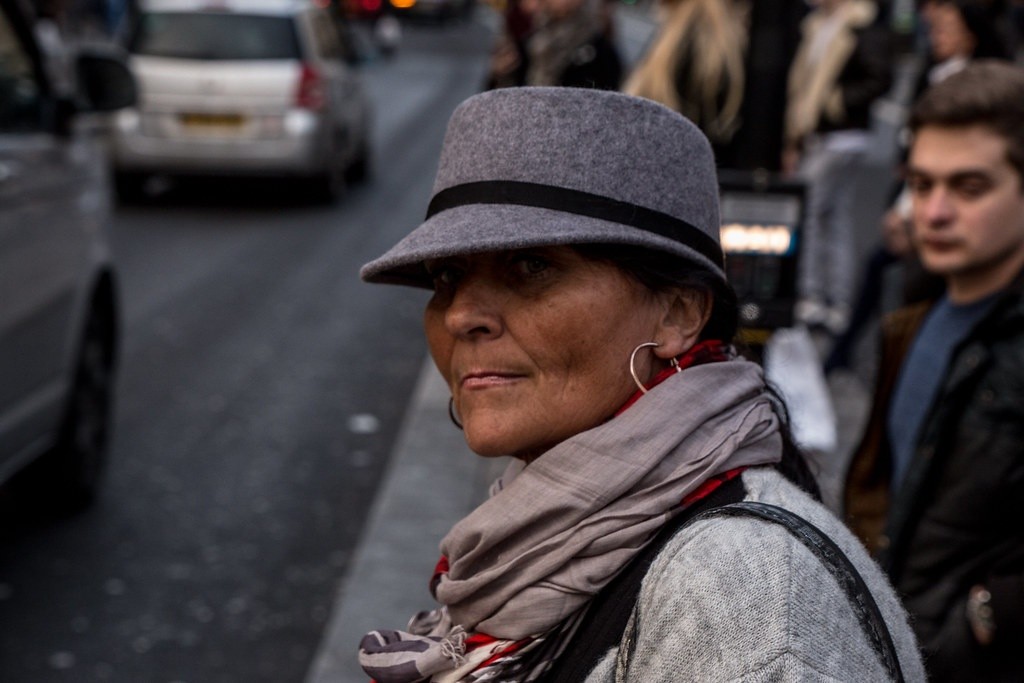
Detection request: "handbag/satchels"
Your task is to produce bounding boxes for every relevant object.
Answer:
[714,182,808,331]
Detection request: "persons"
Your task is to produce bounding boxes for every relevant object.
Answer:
[478,0,1024,454]
[843,63,1024,683]
[356,87,929,683]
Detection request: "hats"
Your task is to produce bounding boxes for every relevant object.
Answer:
[359,84,729,294]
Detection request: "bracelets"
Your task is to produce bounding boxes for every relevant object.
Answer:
[972,589,994,632]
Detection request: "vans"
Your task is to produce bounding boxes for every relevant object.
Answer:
[93,0,376,209]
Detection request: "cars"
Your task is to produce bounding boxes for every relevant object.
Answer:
[0,0,146,526]
